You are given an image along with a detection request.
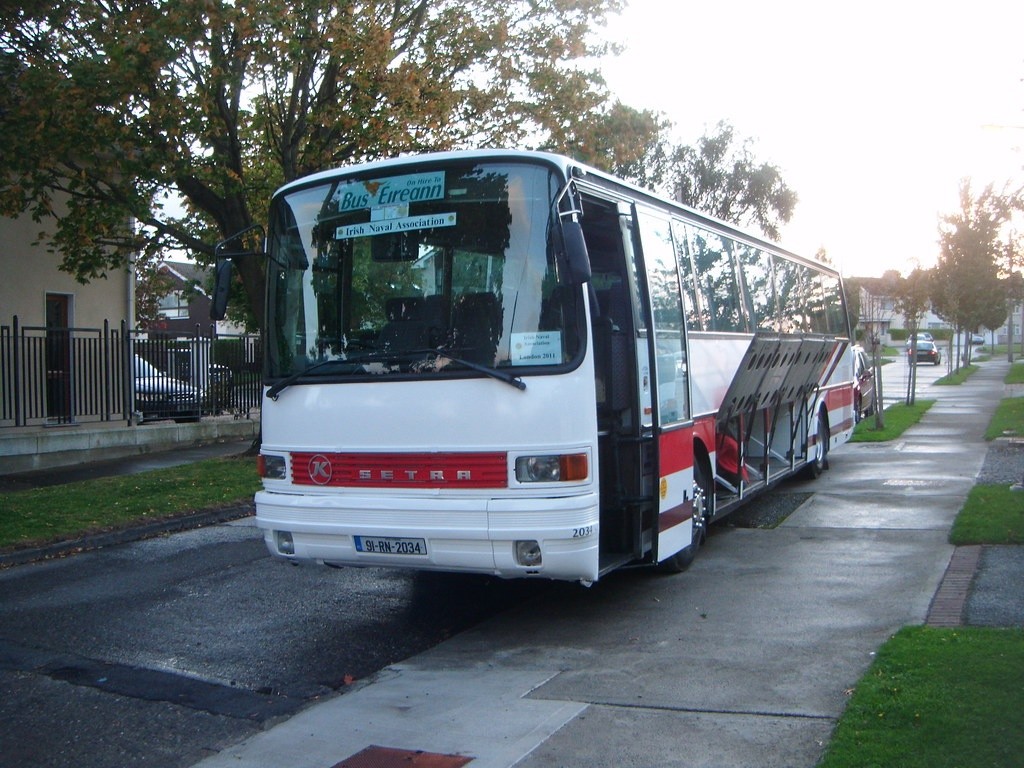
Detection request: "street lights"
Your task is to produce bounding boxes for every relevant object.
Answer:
[1007,226,1018,362]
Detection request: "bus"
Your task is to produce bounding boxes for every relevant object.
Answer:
[210,147,856,589]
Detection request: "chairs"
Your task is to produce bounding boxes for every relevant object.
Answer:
[377,281,577,360]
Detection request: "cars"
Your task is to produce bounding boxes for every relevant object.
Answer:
[906,332,934,350]
[972,334,985,345]
[135,352,203,412]
[907,340,942,365]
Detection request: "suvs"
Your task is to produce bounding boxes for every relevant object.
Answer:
[854,341,878,422]
